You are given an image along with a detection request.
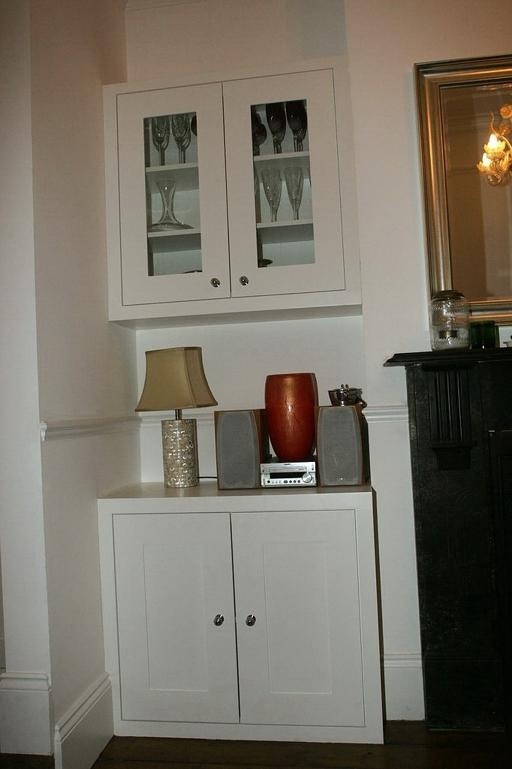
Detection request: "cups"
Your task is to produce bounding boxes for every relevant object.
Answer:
[469,320,501,350]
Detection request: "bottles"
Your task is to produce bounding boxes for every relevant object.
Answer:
[428,289,469,351]
[148,181,194,233]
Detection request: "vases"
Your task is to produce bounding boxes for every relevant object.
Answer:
[266,374,318,460]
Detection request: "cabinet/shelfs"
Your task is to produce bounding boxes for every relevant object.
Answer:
[102,55,364,322]
[98,481,385,746]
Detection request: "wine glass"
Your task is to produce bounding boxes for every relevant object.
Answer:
[252,99,307,223]
[152,114,189,166]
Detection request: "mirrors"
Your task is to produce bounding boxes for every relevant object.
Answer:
[413,54,512,328]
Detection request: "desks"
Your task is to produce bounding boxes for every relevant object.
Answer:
[384,345,510,733]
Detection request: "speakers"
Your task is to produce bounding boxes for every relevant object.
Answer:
[214,409,269,490]
[316,406,370,485]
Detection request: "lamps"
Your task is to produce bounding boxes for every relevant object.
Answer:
[135,347,219,490]
[477,103,512,187]
